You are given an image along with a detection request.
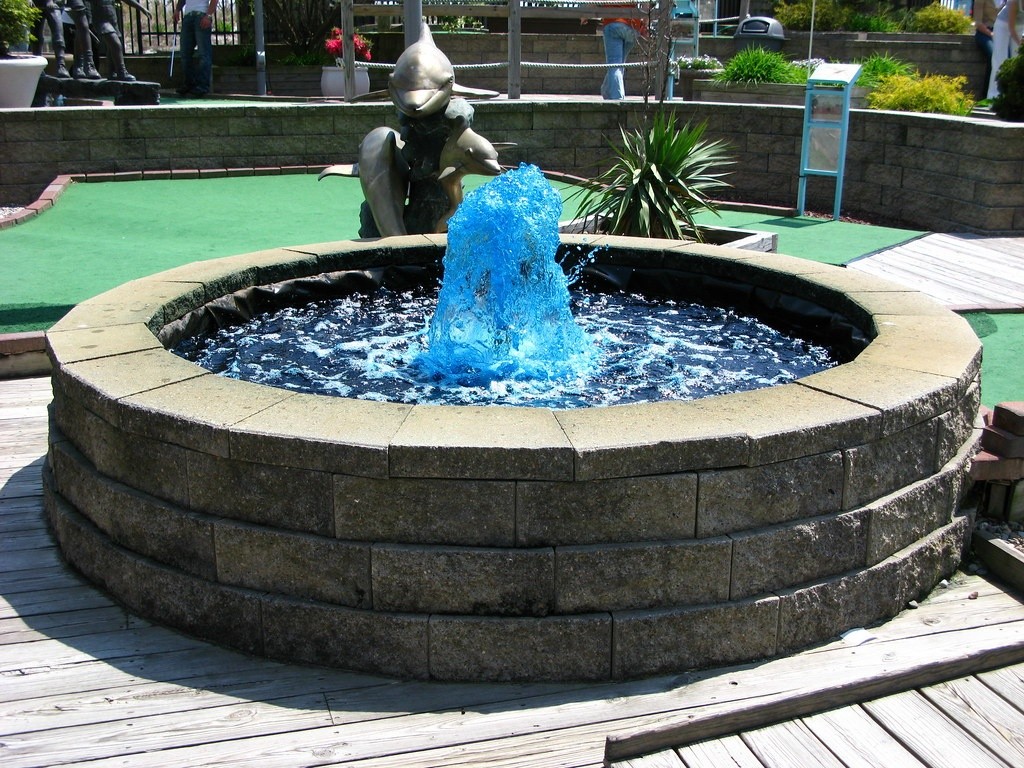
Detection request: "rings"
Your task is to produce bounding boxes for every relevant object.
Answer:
[208,26,210,27]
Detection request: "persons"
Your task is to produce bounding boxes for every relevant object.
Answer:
[974,0,1024,100]
[580,2,650,102]
[27,0,152,81]
[172,0,218,98]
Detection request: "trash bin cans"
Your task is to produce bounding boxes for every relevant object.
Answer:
[733,16,784,62]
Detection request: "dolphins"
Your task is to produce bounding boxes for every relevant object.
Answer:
[316,13,501,236]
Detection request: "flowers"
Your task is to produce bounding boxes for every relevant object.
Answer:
[676,52,723,70]
[324,26,373,61]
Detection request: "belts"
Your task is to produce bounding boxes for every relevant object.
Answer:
[188,11,206,17]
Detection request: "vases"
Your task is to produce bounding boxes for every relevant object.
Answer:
[679,68,723,102]
[320,66,370,101]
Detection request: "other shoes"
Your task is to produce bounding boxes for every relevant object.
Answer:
[190,87,208,96]
[176,88,191,95]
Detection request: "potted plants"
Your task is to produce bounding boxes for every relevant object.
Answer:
[0,0,49,108]
[557,103,779,253]
[692,40,912,112]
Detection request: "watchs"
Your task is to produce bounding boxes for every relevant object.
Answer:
[206,13,212,20]
